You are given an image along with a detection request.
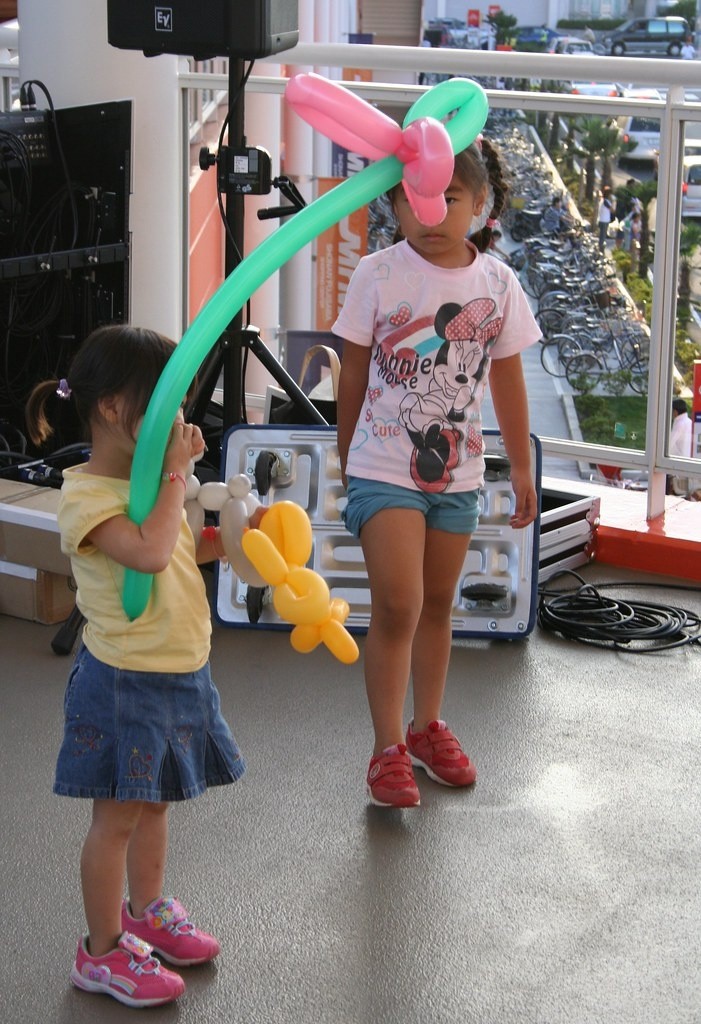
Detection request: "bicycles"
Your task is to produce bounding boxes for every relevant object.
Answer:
[433,74,650,394]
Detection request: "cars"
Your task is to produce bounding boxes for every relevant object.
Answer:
[558,79,699,102]
[653,139,701,180]
[681,156,701,218]
[429,19,598,57]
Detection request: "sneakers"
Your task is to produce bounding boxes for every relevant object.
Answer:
[121,893,220,968]
[405,716,476,787]
[69,930,185,1008]
[366,743,421,807]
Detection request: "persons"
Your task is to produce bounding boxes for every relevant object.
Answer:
[670,399,692,457]
[544,197,582,231]
[616,178,639,249]
[631,212,641,240]
[583,24,596,44]
[26,323,272,1009]
[327,134,542,808]
[681,38,697,59]
[488,230,513,263]
[597,186,615,251]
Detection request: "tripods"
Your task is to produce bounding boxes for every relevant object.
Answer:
[184,55,329,442]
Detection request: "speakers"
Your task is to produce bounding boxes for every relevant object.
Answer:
[106,0,298,62]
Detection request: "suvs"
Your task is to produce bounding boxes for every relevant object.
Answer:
[601,16,693,57]
[613,115,661,167]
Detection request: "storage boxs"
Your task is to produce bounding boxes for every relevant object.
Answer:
[0,479,81,625]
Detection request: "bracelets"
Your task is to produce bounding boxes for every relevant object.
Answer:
[162,471,187,491]
[201,526,228,563]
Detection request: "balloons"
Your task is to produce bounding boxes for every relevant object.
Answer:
[122,74,489,626]
[180,450,362,664]
